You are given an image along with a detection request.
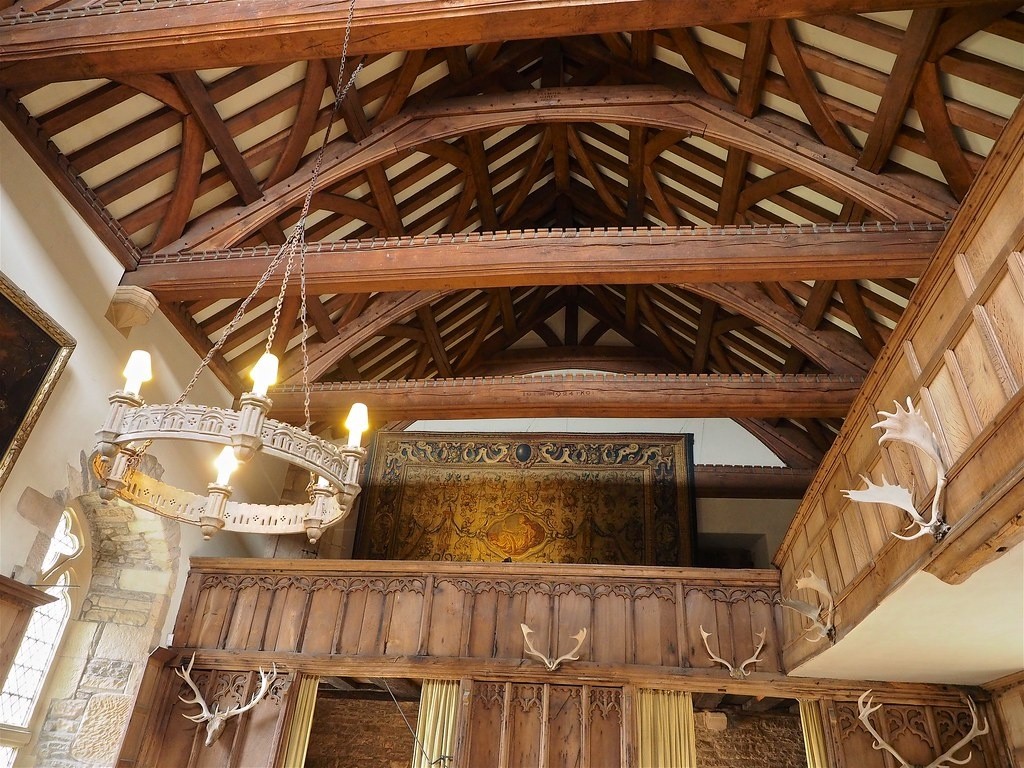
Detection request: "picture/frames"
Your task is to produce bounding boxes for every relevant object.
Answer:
[0,267,77,491]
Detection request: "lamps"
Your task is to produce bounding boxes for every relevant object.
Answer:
[91,0,371,545]
